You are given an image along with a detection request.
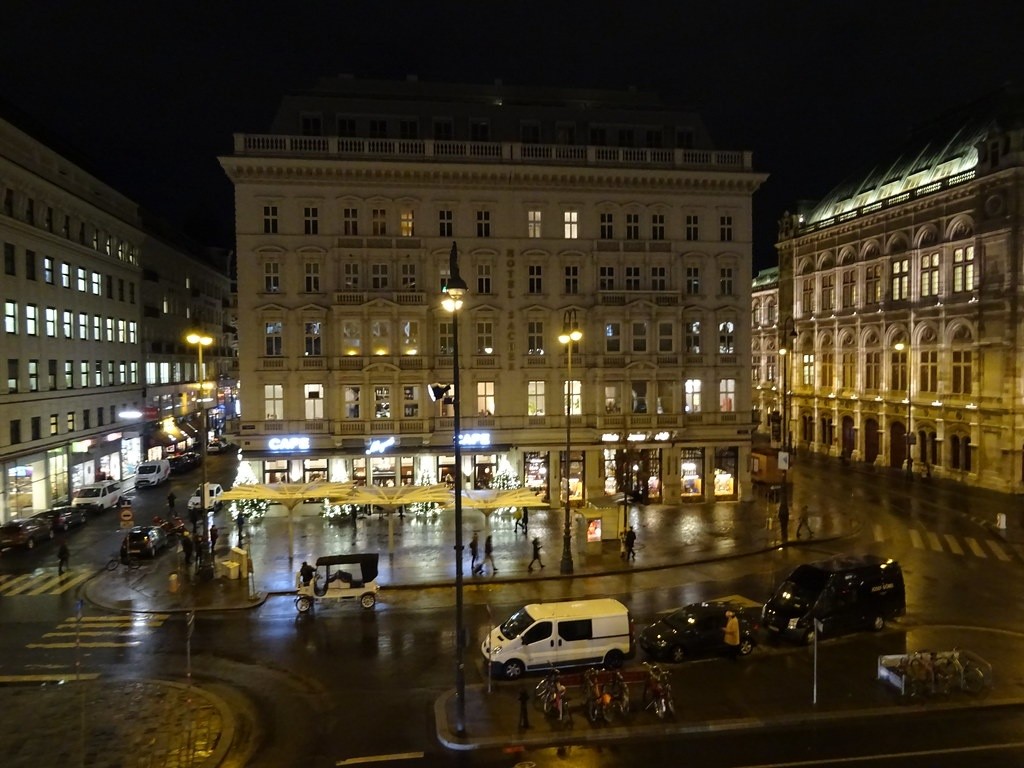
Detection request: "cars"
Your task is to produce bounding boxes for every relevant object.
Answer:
[187,482,224,512]
[639,600,765,663]
[166,452,202,474]
[0,505,86,550]
[120,525,169,561]
[207,441,229,455]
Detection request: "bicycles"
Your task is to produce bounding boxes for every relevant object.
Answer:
[641,659,677,719]
[107,551,143,571]
[533,659,566,727]
[579,667,632,721]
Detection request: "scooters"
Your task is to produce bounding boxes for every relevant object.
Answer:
[153,513,188,536]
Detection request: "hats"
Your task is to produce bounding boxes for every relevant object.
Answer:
[184,531,190,536]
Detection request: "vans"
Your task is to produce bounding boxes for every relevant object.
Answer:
[134,459,171,488]
[71,479,125,513]
[481,598,632,681]
[763,553,907,646]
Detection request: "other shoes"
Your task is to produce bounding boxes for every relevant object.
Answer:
[796,534,801,537]
[626,557,630,560]
[493,568,498,570]
[541,565,545,568]
[481,570,484,572]
[528,566,532,570]
[810,532,814,535]
[632,553,635,557]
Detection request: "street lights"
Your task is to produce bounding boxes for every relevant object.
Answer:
[777,315,798,530]
[186,308,214,580]
[558,308,583,574]
[440,241,470,645]
[895,330,913,480]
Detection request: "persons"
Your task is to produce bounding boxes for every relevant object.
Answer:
[182,532,192,565]
[237,512,244,538]
[167,492,176,509]
[514,507,528,533]
[528,538,546,570]
[300,562,316,584]
[722,611,742,664]
[350,505,357,529]
[195,540,203,565]
[619,526,637,559]
[470,533,478,569]
[796,505,815,537]
[472,535,497,573]
[210,524,218,553]
[57,543,69,574]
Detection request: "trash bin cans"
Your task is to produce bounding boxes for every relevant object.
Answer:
[169,574,178,593]
[222,561,240,580]
[231,547,248,579]
[996,513,1006,528]
